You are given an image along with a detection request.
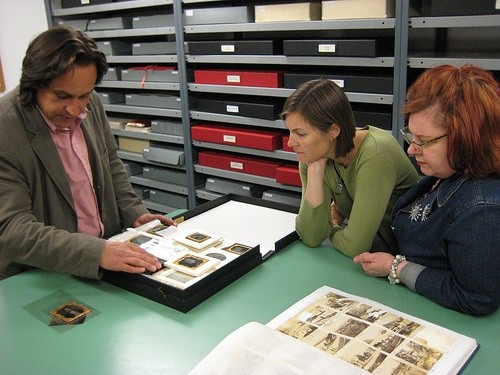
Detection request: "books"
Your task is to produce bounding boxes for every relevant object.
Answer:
[192,286,476,375]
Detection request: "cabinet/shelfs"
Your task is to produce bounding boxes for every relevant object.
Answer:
[44,0,500,213]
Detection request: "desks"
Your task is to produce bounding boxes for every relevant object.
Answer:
[0,208,500,375]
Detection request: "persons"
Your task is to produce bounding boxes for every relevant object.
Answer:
[0,25,178,278]
[280,79,420,259]
[352,64,500,317]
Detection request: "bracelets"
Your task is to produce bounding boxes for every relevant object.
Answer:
[387,255,406,284]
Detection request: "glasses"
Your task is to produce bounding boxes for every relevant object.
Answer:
[399,125,448,155]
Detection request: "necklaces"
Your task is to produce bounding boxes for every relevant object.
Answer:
[333,152,348,195]
